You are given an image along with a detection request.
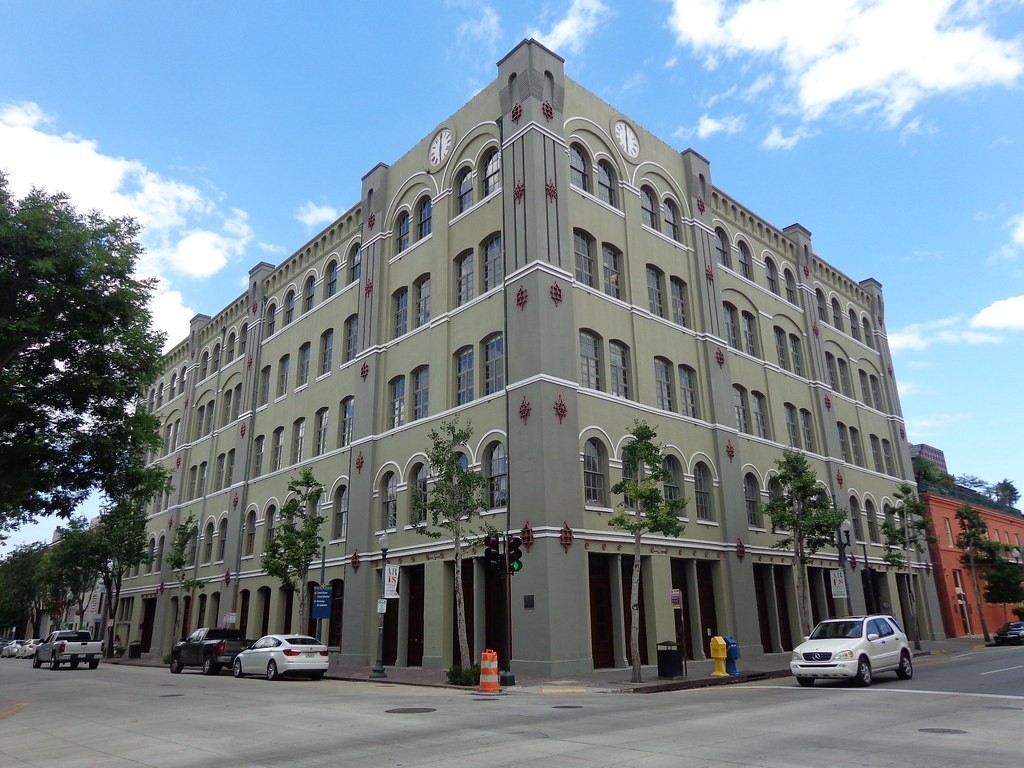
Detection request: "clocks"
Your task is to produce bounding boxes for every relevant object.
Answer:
[609,113,644,165]
[424,122,457,174]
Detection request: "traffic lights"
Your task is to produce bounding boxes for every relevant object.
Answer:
[507,534,522,572]
[484,534,501,572]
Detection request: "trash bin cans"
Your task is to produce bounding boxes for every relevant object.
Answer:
[655,641,683,679]
[723,636,741,676]
[710,636,729,676]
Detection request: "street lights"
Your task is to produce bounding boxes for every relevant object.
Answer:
[827,518,852,616]
[369,529,389,679]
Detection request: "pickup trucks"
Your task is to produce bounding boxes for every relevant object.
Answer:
[170,627,257,674]
[32,630,104,669]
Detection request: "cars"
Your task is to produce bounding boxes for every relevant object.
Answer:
[232,634,329,681]
[16,639,40,658]
[993,621,1024,645]
[1,640,23,658]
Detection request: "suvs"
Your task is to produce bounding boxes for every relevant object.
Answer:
[789,613,913,688]
